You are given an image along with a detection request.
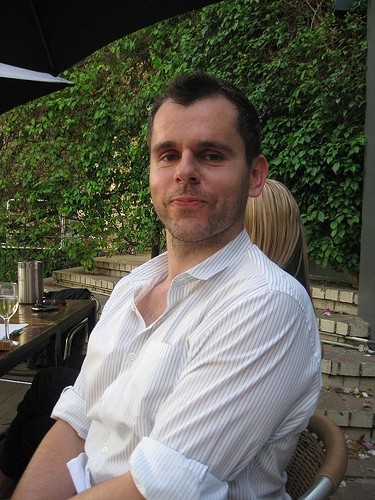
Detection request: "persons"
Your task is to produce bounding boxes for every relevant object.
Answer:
[11,74,323,500]
[0,179,313,481]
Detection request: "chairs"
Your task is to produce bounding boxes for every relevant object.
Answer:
[281,412,348,500]
[22,288,100,368]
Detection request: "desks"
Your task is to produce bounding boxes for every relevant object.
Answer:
[0,297,98,377]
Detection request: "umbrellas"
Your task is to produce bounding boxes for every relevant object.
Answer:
[0,63,75,114]
[0,0,224,78]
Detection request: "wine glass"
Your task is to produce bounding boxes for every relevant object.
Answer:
[0,282,20,346]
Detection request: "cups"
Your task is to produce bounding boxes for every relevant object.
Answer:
[18,261,43,304]
